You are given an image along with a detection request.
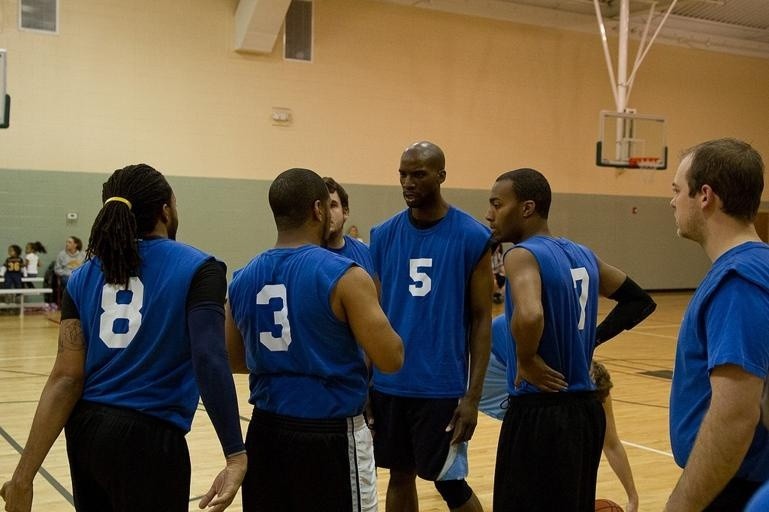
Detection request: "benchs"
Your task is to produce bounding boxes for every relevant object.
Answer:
[1,277,53,315]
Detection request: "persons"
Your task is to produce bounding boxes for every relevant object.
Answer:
[321,176,382,362]
[467,308,640,512]
[345,225,365,245]
[1,244,27,299]
[0,164,248,511]
[659,137,768,511]
[225,167,404,512]
[53,236,87,298]
[364,140,498,512]
[489,245,508,300]
[486,166,657,512]
[15,240,47,298]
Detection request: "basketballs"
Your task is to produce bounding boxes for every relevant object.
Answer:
[595,499,623,512]
